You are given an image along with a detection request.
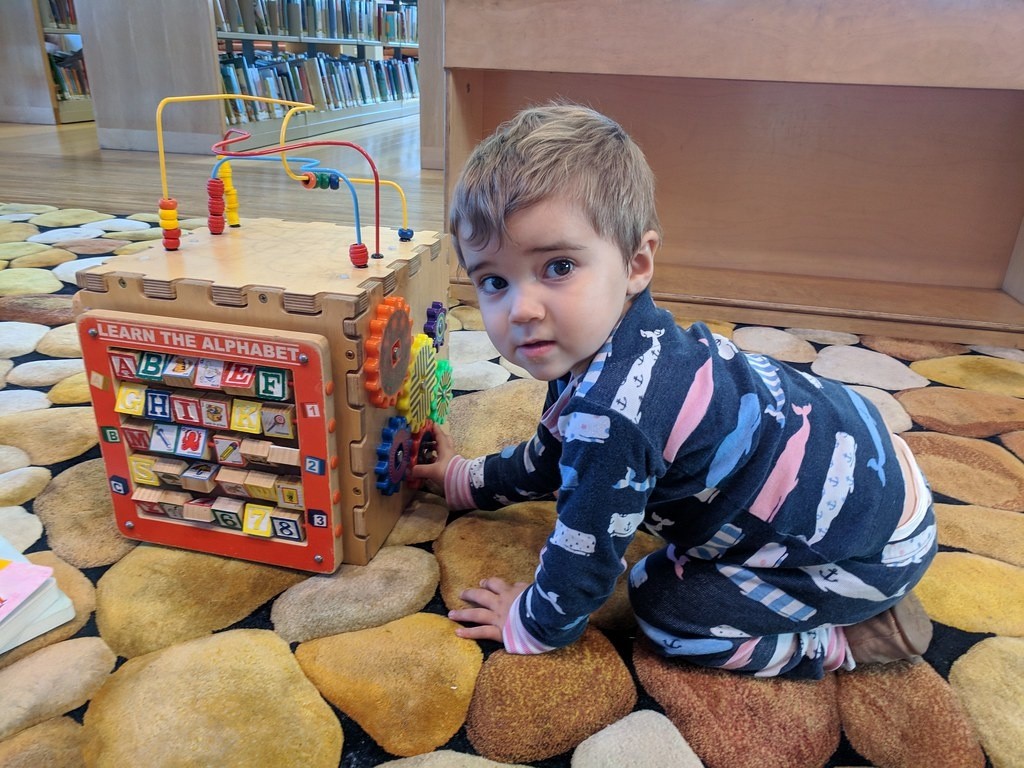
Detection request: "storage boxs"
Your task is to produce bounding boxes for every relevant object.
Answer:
[75,215,453,576]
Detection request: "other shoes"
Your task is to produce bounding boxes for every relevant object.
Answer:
[843,591,933,662]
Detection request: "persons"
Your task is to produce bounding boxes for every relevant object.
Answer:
[412,103,939,682]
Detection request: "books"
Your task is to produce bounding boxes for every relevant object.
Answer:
[37,0,77,29]
[47,47,91,100]
[217,49,420,125]
[0,536,76,655]
[212,0,418,45]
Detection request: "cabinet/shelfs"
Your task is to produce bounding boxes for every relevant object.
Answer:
[1,1,1024,350]
